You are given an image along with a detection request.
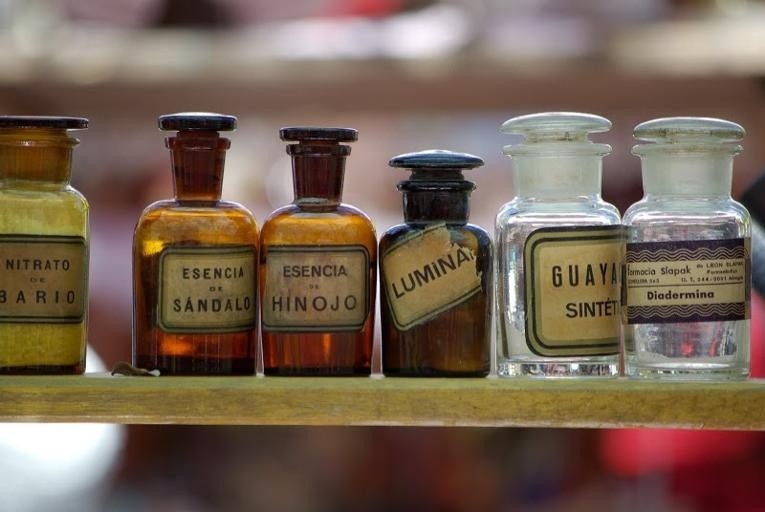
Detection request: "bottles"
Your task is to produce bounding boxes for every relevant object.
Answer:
[0,115,92,376]
[624,117,753,379]
[131,114,258,376]
[492,111,623,377]
[257,125,378,376]
[380,151,492,375]
